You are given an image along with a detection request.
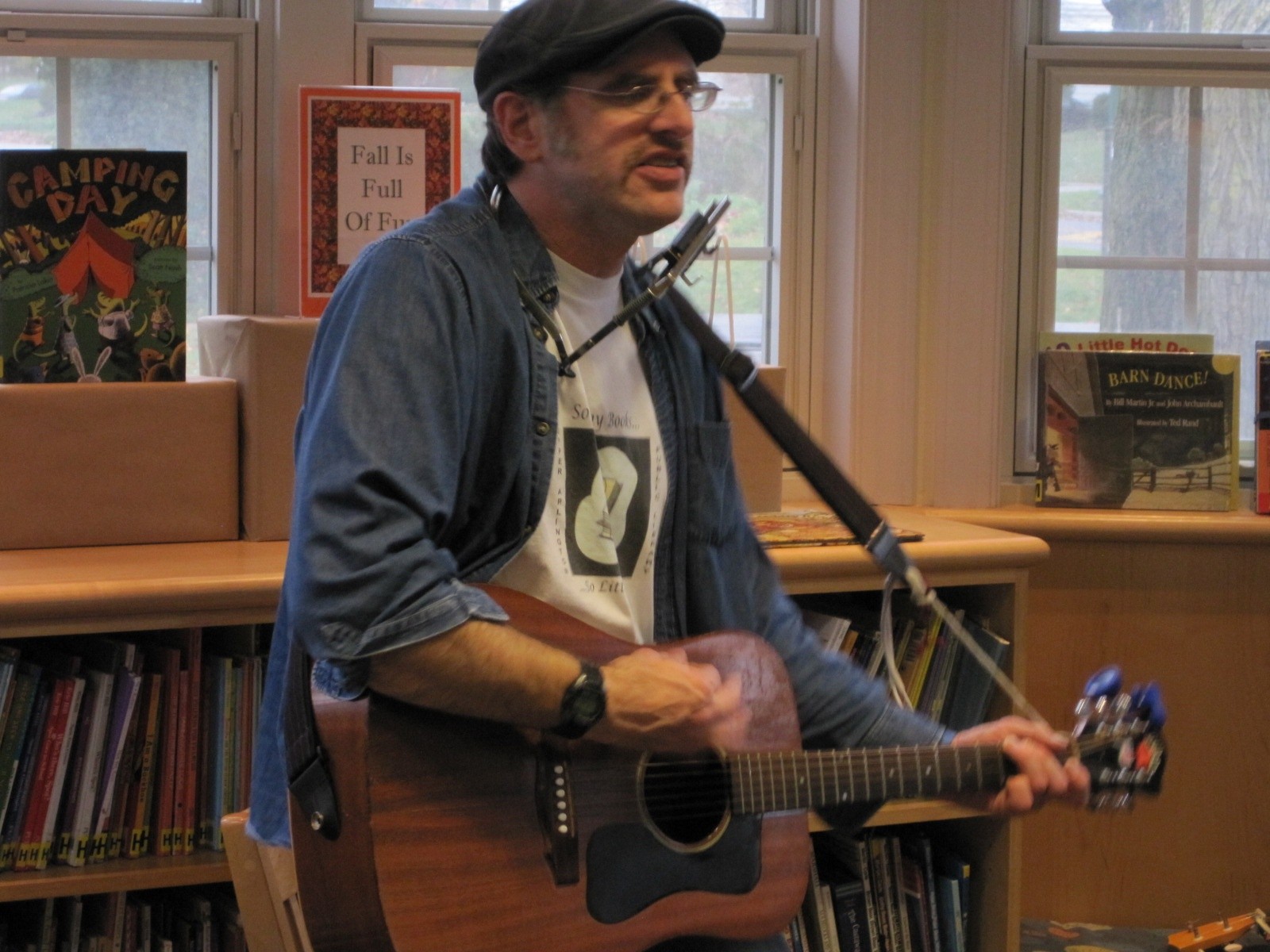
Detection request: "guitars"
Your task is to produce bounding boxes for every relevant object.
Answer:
[286,581,1172,952]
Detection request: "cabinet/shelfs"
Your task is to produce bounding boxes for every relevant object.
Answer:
[2,509,1047,952]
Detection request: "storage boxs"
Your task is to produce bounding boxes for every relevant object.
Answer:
[1,373,239,549]
[1035,332,1242,512]
[196,315,322,542]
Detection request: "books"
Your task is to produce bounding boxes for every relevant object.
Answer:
[799,602,1011,730]
[1035,330,1270,514]
[0,639,271,952]
[781,835,976,952]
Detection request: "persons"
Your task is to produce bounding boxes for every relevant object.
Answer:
[246,0,1092,952]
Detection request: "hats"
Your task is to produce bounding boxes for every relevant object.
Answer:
[474,0,725,108]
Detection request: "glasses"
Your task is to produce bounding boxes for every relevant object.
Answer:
[562,81,720,114]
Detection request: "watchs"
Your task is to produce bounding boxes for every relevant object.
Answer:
[539,655,607,739]
